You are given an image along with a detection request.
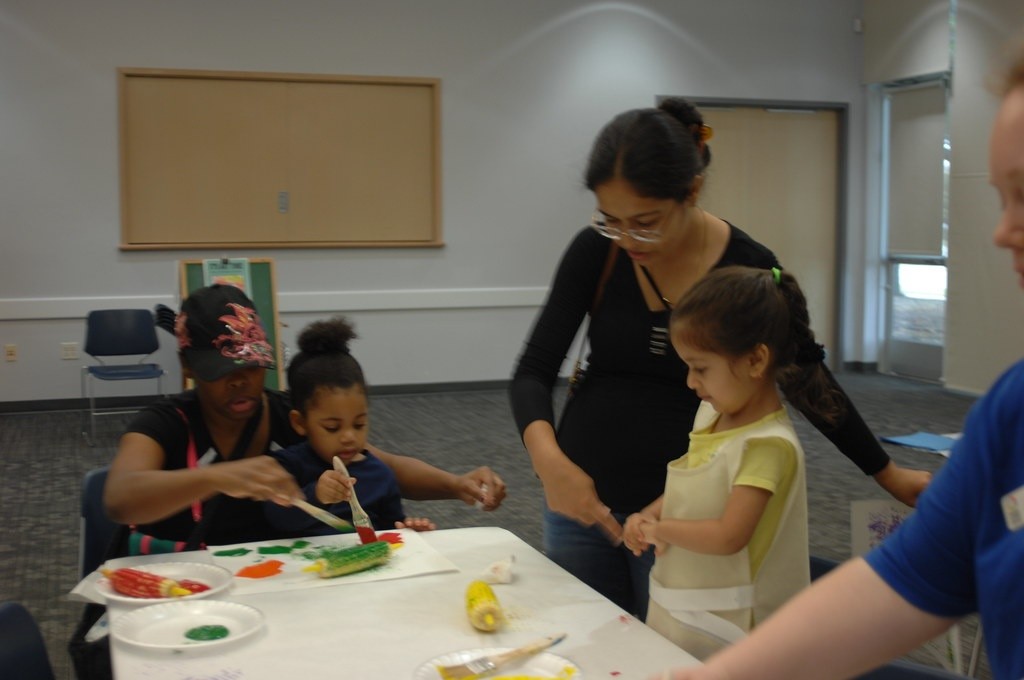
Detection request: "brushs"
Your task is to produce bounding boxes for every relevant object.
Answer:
[289,498,357,536]
[331,456,377,546]
[438,631,568,680]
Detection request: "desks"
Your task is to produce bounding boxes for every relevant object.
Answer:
[105,527,705,680]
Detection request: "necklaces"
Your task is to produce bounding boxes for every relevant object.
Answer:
[662,205,707,305]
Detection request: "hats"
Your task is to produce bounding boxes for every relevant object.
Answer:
[175,284,275,381]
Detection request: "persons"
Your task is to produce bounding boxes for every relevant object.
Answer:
[267,320,435,537]
[98,284,505,553]
[509,98,931,624]
[659,46,1024,680]
[625,267,812,662]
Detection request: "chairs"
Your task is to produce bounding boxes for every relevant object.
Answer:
[76,465,117,584]
[79,309,167,446]
[0,602,55,680]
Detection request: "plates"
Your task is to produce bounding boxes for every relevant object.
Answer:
[94,561,232,606]
[111,599,264,651]
[414,646,582,680]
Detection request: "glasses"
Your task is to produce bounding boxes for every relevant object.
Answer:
[591,183,684,242]
